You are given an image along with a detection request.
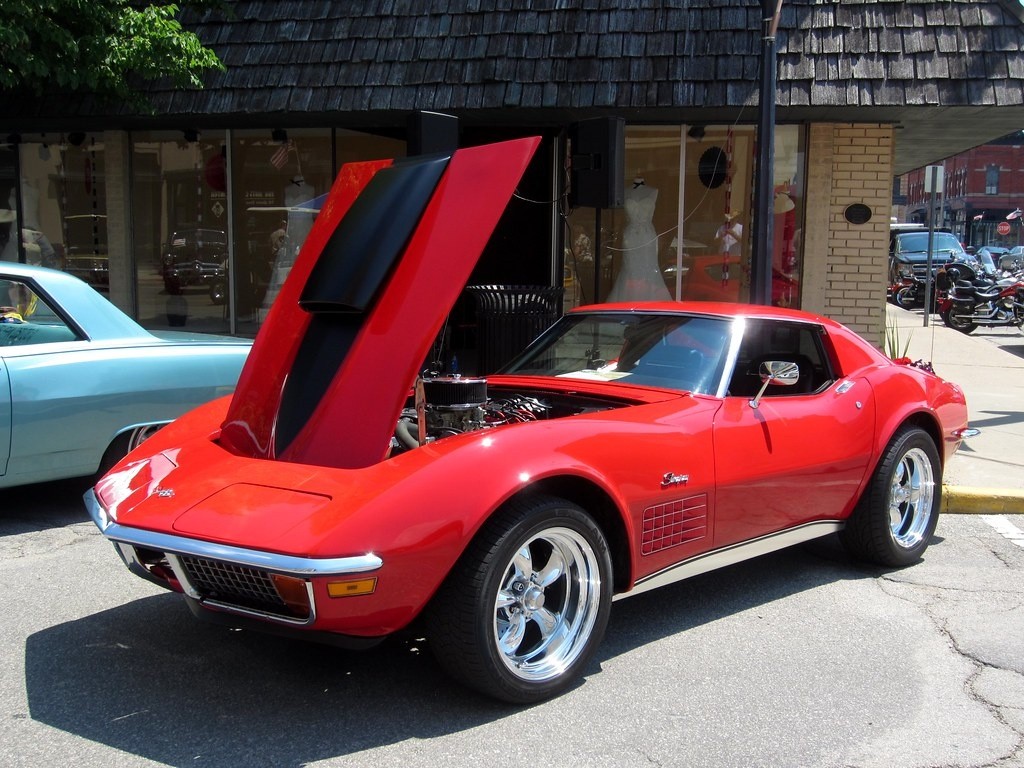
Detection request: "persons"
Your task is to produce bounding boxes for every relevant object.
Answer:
[269,220,287,256]
[33,230,54,268]
[261,175,314,322]
[606,178,677,302]
[715,218,743,255]
[772,190,795,271]
[572,225,593,270]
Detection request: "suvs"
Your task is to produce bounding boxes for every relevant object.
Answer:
[890,231,978,305]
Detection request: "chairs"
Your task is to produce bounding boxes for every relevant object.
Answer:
[631,343,706,385]
[745,351,815,397]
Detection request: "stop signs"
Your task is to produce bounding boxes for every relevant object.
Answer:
[997,221,1012,235]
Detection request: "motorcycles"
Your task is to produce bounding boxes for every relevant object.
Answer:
[890,248,1024,336]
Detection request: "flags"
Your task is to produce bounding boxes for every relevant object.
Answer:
[271,138,297,170]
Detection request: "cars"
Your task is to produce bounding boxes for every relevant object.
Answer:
[997,246,1024,272]
[159,227,229,305]
[973,245,1010,268]
[0,260,253,493]
[660,254,798,308]
[65,214,110,291]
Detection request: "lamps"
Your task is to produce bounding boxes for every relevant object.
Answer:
[271,128,288,144]
[184,128,199,143]
[687,125,706,140]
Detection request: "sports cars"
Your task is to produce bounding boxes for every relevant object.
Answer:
[81,134,979,708]
